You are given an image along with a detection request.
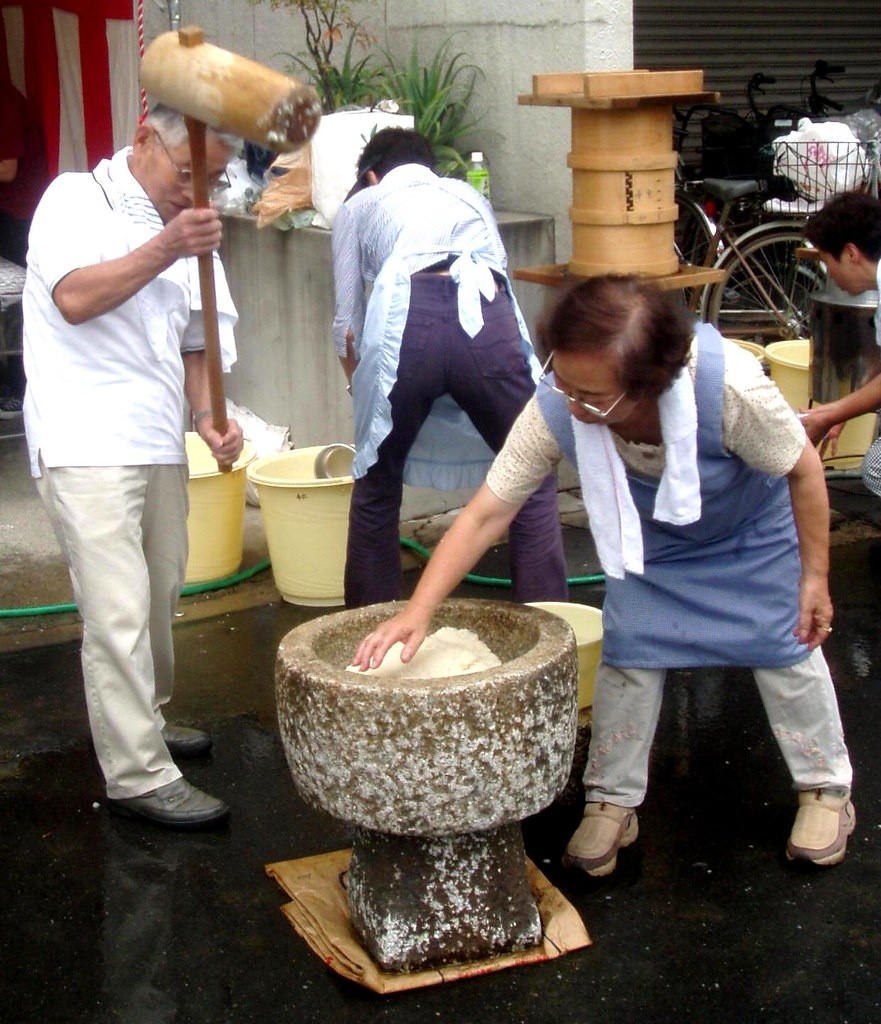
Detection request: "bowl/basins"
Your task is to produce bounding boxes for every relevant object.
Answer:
[315,443,355,478]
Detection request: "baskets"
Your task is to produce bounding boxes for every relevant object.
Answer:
[762,141,873,216]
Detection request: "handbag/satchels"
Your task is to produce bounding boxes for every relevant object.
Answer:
[701,112,759,181]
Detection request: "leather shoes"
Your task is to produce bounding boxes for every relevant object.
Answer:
[107,778,230,828]
[162,723,214,756]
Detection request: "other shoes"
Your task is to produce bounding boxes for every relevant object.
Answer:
[0,385,13,404]
[564,801,638,876]
[786,788,856,864]
[0,397,23,419]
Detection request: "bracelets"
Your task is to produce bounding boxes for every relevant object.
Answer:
[194,410,213,435]
[346,385,353,398]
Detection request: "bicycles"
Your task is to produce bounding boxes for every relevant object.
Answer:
[669,59,881,374]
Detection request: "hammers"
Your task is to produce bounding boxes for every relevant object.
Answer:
[138,24,321,473]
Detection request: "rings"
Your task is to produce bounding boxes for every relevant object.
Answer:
[815,625,833,633]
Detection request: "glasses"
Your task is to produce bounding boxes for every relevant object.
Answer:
[539,351,627,419]
[152,127,231,196]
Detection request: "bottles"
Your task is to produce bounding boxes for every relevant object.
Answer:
[467,152,490,203]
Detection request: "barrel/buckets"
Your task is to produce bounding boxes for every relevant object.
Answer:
[524,602,602,711]
[809,291,881,414]
[247,444,354,606]
[185,431,255,583]
[765,340,877,469]
[728,339,764,360]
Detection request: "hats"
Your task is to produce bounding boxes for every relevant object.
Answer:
[343,154,383,202]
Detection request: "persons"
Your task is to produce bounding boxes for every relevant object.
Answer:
[19,101,242,823]
[796,191,881,500]
[0,79,49,421]
[350,273,857,876]
[331,126,570,611]
